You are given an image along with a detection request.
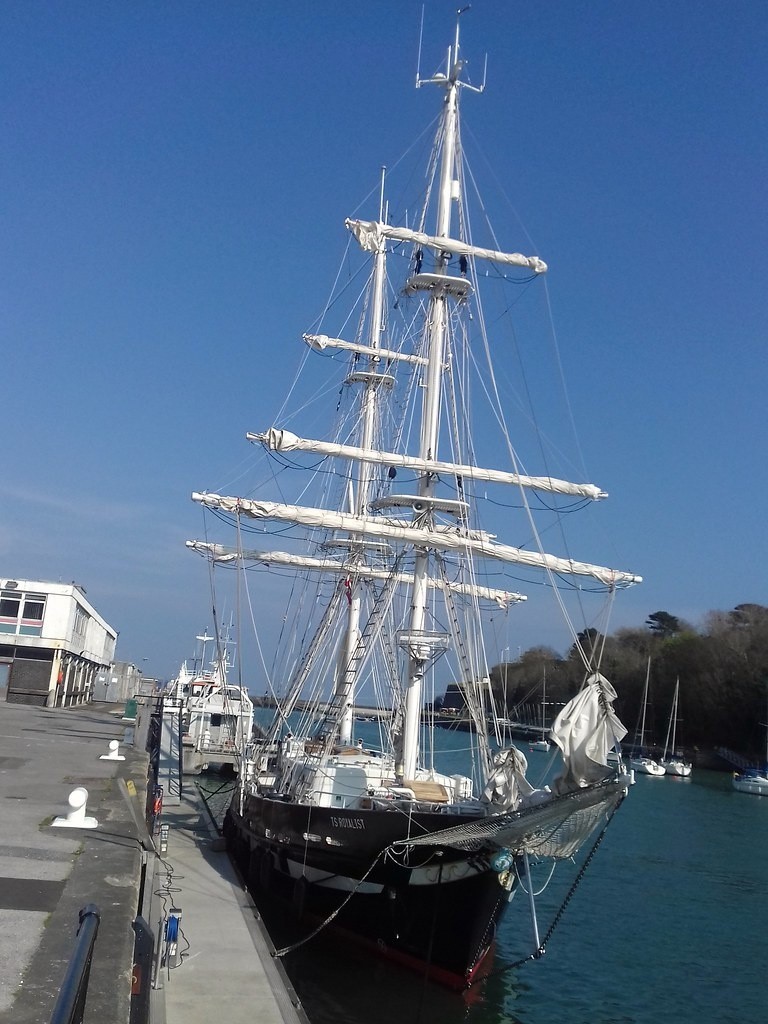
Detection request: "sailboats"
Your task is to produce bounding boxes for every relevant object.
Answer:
[627,655,668,778]
[526,663,550,753]
[656,674,694,776]
[180,11,648,998]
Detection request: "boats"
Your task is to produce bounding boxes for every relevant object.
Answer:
[731,768,768,796]
